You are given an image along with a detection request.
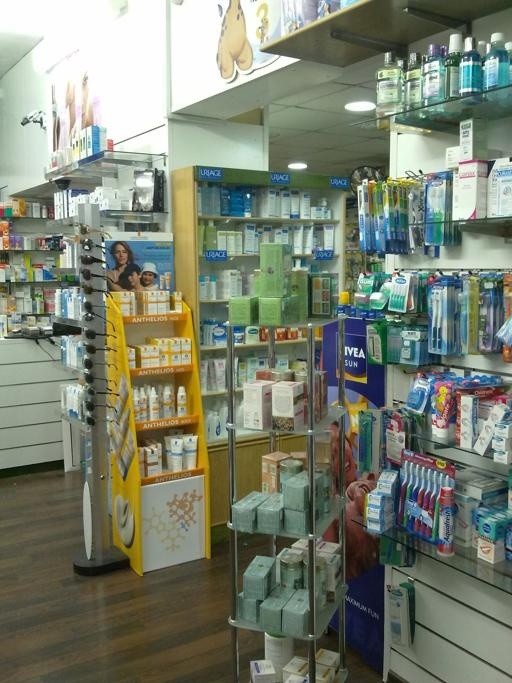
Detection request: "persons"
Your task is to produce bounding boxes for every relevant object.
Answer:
[124,263,147,290]
[141,260,160,290]
[321,420,361,583]
[341,477,380,556]
[106,241,133,290]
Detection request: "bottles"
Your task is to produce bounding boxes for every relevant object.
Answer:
[208,274,217,300]
[131,382,187,422]
[374,32,511,124]
[436,486,458,557]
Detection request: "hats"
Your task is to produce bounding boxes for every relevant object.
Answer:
[122,264,140,277]
[140,263,158,278]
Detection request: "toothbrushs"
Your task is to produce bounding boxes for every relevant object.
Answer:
[431,281,512,359]
[397,460,451,550]
[358,187,461,244]
